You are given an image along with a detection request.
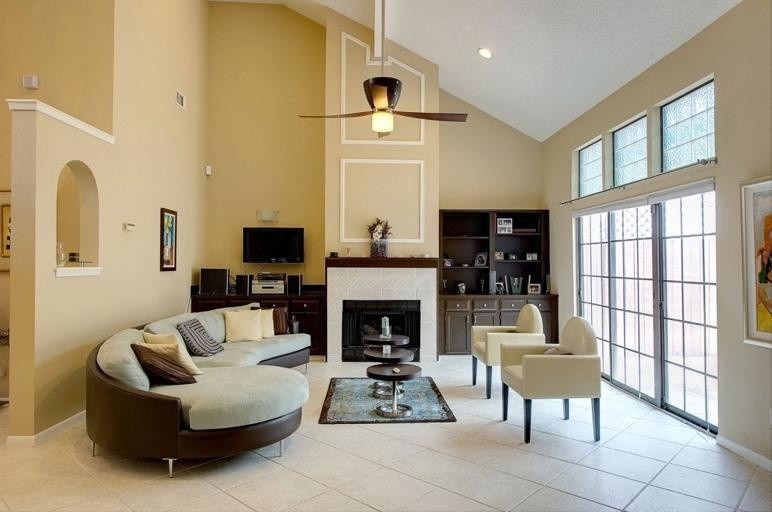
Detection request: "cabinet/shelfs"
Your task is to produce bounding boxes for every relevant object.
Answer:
[191,294,327,362]
[439,209,558,355]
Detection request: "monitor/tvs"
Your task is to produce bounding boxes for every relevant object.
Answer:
[242,227,304,264]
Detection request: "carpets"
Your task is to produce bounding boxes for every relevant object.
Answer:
[319,377,457,424]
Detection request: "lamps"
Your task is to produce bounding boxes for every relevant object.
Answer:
[372,112,394,133]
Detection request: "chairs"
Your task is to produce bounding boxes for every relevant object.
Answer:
[501,316,601,444]
[470,304,546,400]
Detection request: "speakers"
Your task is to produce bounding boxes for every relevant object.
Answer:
[200,268,230,297]
[235,274,253,297]
[286,274,303,296]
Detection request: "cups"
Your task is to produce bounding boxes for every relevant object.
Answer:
[383,345,391,355]
[510,273,523,293]
[57,243,65,267]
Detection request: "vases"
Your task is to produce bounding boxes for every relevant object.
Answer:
[371,243,387,257]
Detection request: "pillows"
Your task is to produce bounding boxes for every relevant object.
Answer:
[131,307,291,384]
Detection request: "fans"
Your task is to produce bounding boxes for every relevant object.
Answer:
[299,0,468,138]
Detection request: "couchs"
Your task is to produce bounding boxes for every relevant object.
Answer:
[86,302,312,478]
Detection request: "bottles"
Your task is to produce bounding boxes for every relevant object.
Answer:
[381,316,389,336]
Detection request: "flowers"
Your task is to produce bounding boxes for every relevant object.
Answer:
[367,218,393,245]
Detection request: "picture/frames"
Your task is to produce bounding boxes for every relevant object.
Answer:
[497,218,513,235]
[0,190,9,271]
[738,175,772,350]
[528,283,541,294]
[160,207,176,271]
